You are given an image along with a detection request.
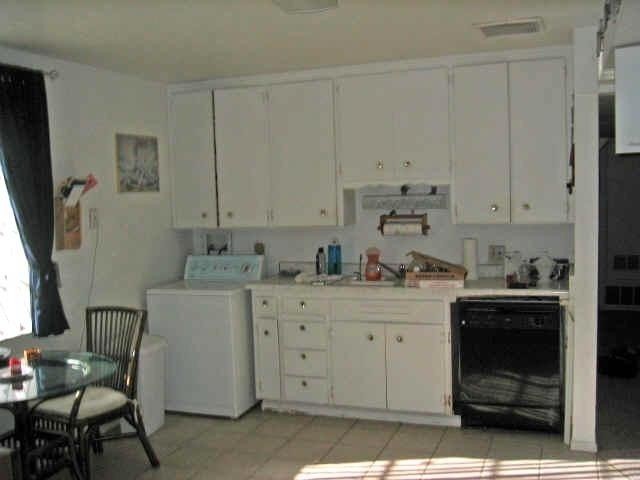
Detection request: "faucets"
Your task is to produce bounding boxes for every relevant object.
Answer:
[399,264,406,279]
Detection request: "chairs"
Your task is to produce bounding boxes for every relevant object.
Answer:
[35,308,160,476]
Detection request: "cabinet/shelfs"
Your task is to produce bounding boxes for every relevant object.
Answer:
[279,295,330,405]
[267,79,337,226]
[252,295,280,400]
[453,56,568,225]
[337,68,451,186]
[330,300,460,415]
[165,86,267,228]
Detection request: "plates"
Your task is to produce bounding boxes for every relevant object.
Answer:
[350,275,398,286]
[2,367,33,378]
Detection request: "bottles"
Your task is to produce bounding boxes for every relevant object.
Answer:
[519,258,531,285]
[316,247,325,276]
[366,247,381,281]
[327,241,342,276]
